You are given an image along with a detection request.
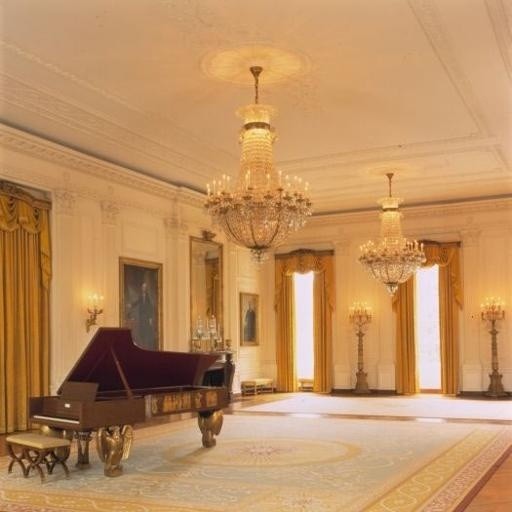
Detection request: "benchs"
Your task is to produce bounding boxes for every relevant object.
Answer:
[242,378,274,397]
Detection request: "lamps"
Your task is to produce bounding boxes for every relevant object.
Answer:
[205,67,313,263]
[479,294,508,397]
[349,301,373,393]
[357,172,427,297]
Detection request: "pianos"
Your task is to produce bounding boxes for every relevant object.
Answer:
[29,327,235,478]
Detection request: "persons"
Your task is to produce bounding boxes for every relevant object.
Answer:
[126,281,156,351]
[244,301,256,340]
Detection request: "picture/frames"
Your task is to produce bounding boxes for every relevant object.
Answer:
[118,256,163,353]
[240,292,260,345]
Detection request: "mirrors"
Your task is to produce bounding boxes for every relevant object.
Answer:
[189,237,225,354]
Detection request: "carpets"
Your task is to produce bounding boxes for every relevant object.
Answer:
[1,395,512,512]
